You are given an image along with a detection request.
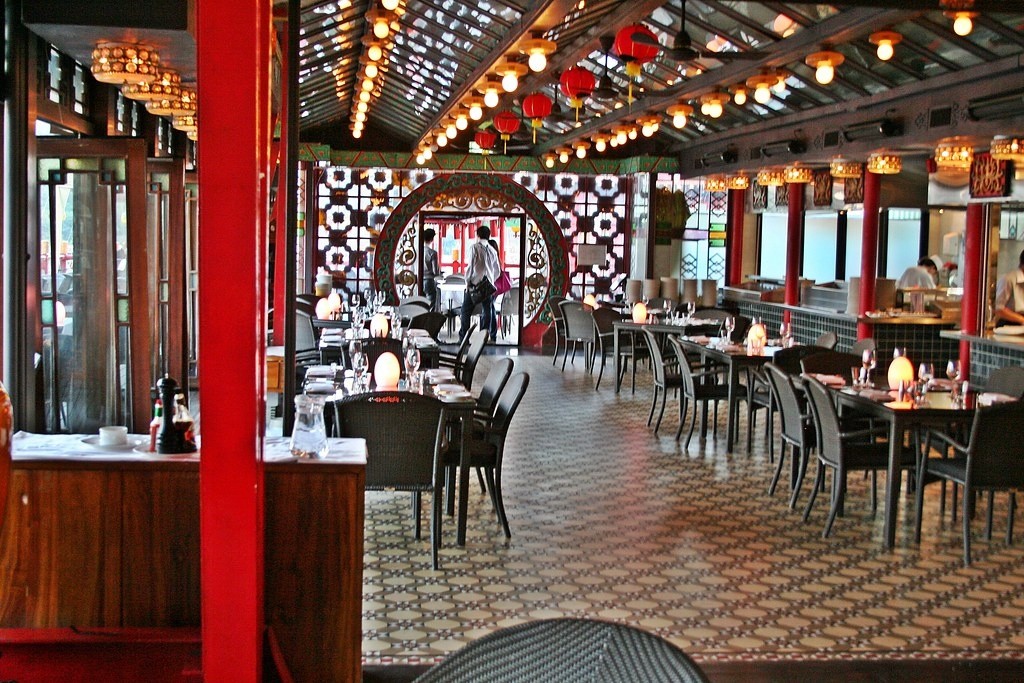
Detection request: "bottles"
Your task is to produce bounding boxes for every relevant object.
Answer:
[149,386,197,454]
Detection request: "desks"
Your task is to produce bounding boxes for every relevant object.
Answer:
[312,314,413,338]
[592,306,667,320]
[299,368,477,548]
[789,374,1019,549]
[318,327,441,369]
[340,305,394,313]
[612,318,686,394]
[436,284,506,339]
[677,336,784,454]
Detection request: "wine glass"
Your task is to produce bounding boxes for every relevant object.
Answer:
[622,293,962,407]
[348,289,424,394]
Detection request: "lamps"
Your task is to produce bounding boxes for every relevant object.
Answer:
[584,294,595,306]
[887,357,914,391]
[328,293,340,312]
[369,313,388,338]
[373,352,401,392]
[88,0,1024,194]
[748,324,766,348]
[895,268,936,313]
[571,141,591,159]
[633,303,646,324]
[315,298,329,320]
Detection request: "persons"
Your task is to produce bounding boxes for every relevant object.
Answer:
[896,255,944,302]
[996,249,1024,328]
[410,229,441,312]
[458,226,502,341]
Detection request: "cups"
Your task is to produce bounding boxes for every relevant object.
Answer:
[99,426,128,445]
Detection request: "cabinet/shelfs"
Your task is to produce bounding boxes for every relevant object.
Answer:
[0,433,366,683]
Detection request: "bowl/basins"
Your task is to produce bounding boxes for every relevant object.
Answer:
[305,327,342,393]
[408,329,472,402]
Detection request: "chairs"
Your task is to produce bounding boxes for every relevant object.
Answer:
[34,333,73,426]
[547,295,1024,564]
[296,273,529,571]
[413,617,711,683]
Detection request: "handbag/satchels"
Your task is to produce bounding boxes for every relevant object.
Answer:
[469,277,497,306]
[496,269,511,296]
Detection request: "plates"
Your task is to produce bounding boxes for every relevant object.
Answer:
[132,444,200,458]
[80,437,148,451]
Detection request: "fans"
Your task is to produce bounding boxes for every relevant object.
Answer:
[500,68,601,123]
[472,94,568,141]
[612,0,773,63]
[547,33,675,99]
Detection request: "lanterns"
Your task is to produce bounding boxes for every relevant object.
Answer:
[523,91,553,143]
[494,109,520,154]
[474,129,497,170]
[560,66,595,121]
[614,24,659,105]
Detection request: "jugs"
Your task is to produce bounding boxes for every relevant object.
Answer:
[290,394,328,460]
